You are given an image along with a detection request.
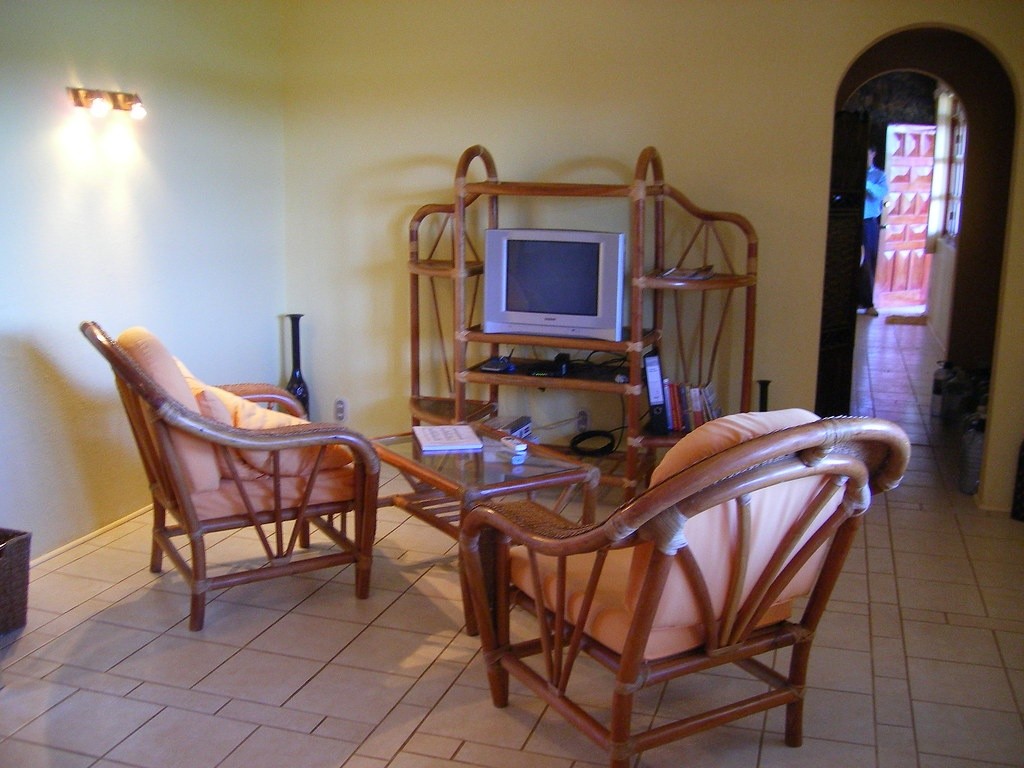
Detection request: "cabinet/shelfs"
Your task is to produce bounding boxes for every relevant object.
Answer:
[407,145,760,507]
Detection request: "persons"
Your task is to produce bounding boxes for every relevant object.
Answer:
[853,139,890,318]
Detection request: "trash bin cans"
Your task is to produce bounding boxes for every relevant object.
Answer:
[0,527,33,636]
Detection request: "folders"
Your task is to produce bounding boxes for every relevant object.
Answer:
[643,350,668,436]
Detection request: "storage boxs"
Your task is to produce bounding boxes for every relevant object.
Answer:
[0,527,31,635]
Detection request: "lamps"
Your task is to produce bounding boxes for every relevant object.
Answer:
[67,87,149,123]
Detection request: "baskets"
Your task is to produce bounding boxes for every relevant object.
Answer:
[0,527,31,634]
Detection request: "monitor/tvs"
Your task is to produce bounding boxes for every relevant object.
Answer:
[483,228,624,342]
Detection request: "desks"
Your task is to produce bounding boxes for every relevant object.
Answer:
[353,422,601,638]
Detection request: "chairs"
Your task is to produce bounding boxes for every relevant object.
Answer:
[457,408,914,766]
[79,320,381,632]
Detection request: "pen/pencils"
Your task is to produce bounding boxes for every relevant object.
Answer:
[663,267,676,277]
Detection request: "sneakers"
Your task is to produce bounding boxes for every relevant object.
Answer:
[866,306,878,316]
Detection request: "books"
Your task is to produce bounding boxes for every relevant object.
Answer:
[661,376,719,435]
[655,265,717,281]
[411,425,483,452]
